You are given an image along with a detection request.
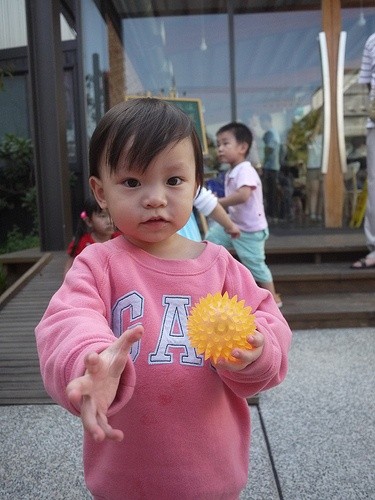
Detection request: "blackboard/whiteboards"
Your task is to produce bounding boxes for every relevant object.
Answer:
[125,96,208,155]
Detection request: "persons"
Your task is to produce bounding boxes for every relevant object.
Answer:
[63,203,123,283]
[352,30,375,268]
[177,108,327,308]
[35,98,294,500]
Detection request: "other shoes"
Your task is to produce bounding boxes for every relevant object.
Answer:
[350,258,375,269]
